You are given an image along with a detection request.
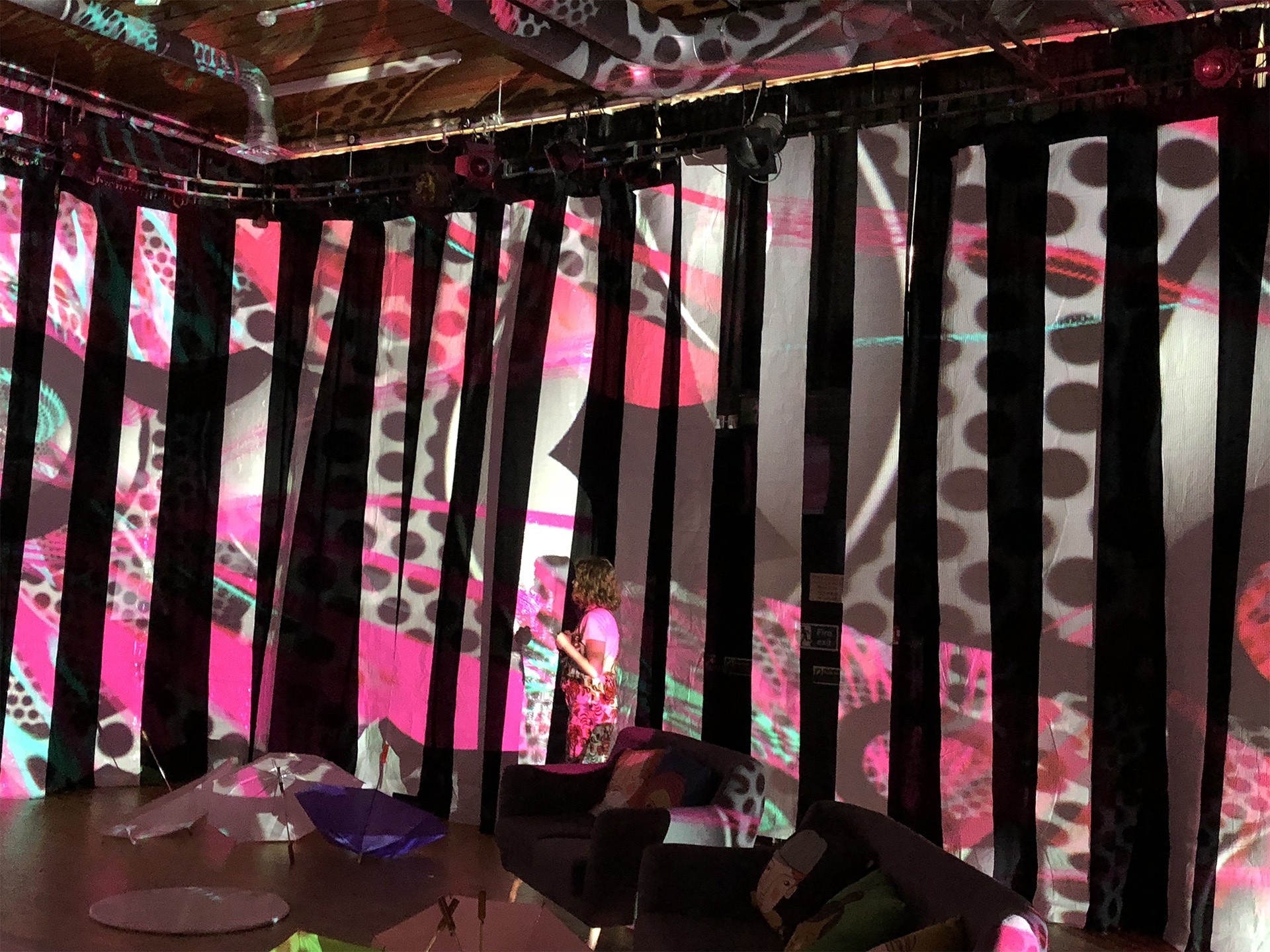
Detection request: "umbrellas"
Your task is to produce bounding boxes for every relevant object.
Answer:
[96,729,239,845]
[269,927,385,952]
[371,890,593,952]
[294,744,450,865]
[208,753,365,865]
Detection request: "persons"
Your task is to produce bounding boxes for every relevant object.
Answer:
[556,555,620,766]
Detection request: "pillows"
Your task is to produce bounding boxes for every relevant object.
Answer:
[592,746,686,824]
[751,826,838,938]
[784,868,914,952]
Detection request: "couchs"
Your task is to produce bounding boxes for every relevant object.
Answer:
[494,728,769,923]
[627,800,1045,952]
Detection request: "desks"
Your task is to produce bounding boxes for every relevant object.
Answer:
[0,782,594,952]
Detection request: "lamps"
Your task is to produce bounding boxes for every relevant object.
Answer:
[60,87,798,216]
[1193,47,1244,90]
